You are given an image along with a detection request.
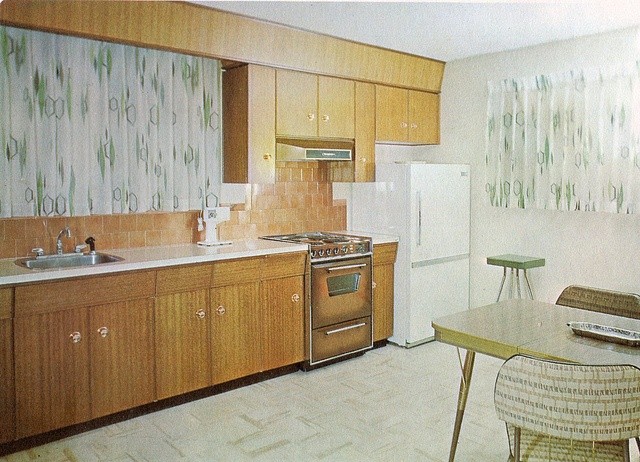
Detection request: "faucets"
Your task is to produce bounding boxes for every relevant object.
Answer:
[55,225,72,255]
[84,236,96,254]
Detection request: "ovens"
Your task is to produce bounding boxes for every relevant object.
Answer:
[307,255,374,365]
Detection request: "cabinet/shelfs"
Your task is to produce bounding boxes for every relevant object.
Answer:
[351,81,375,183]
[373,244,394,350]
[270,69,355,145]
[15,266,156,450]
[1,287,15,456]
[246,66,275,184]
[153,256,266,409]
[261,253,306,381]
[372,84,439,145]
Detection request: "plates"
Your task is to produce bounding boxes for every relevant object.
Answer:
[567,319,640,346]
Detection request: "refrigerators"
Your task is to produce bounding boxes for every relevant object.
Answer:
[376,161,471,348]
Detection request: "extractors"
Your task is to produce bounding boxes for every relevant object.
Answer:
[277,139,354,163]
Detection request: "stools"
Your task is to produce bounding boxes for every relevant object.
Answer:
[486,255,545,303]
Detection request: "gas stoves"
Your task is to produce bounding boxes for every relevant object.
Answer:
[261,230,371,251]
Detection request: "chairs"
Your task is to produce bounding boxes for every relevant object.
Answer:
[494,353,640,462]
[592,402,603,404]
[555,285,639,323]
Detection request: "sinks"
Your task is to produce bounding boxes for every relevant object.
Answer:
[13,251,126,272]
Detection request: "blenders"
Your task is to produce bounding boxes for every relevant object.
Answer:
[196,192,232,248]
